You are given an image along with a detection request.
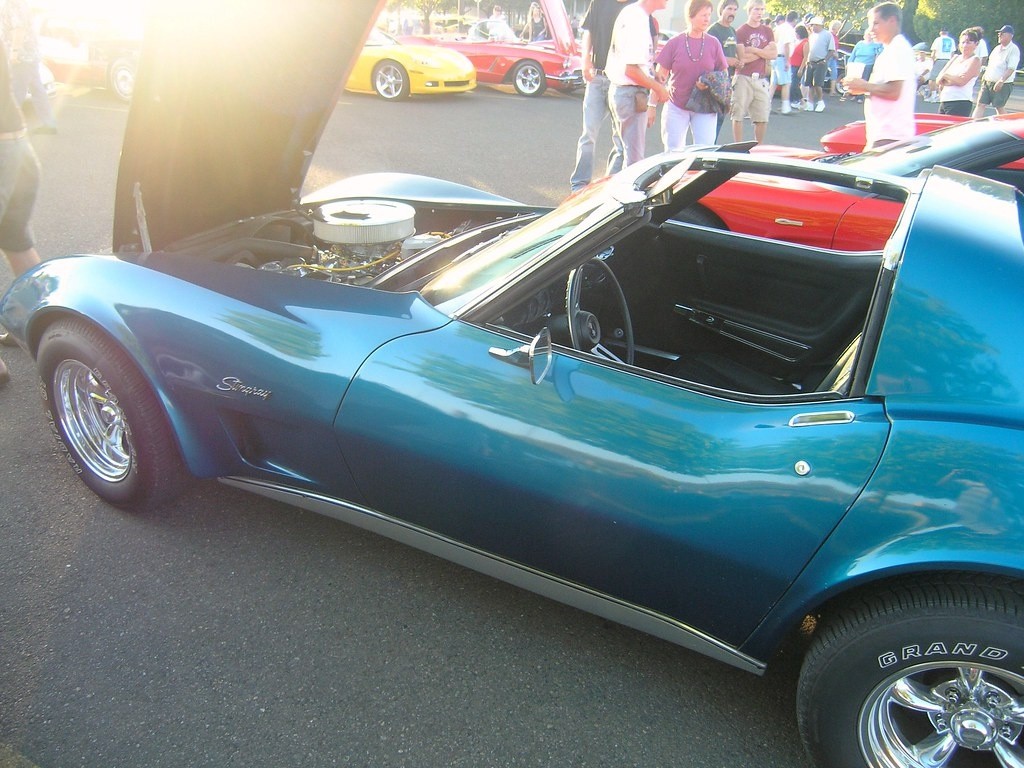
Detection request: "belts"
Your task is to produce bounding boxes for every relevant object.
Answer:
[0,127,28,141]
[593,68,607,77]
[778,54,784,57]
[807,59,825,65]
[745,72,768,80]
[937,59,950,60]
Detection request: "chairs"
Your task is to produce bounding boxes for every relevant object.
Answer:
[665,330,862,398]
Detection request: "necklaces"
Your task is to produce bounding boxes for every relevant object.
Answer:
[686,30,704,62]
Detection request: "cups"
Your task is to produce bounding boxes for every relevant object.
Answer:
[846,61,866,82]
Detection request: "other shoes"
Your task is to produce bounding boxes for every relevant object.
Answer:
[931,96,940,102]
[829,92,836,96]
[804,102,816,112]
[0,332,20,347]
[781,104,792,114]
[791,98,808,110]
[850,96,856,101]
[815,103,825,112]
[924,95,936,102]
[858,99,863,103]
[32,122,57,135]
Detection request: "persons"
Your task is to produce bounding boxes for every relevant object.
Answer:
[0,0,56,384]
[841,3,916,153]
[569,0,778,194]
[487,5,501,42]
[520,2,547,42]
[913,25,1020,118]
[761,11,841,115]
[846,27,884,103]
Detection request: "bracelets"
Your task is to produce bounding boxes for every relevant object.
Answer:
[647,105,656,108]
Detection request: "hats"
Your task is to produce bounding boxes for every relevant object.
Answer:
[803,13,815,24]
[940,27,948,31]
[808,17,823,25]
[995,24,1014,35]
[772,14,785,23]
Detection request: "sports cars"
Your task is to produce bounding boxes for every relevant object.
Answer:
[653,29,673,55]
[824,43,855,96]
[559,111,1024,251]
[395,18,593,97]
[344,26,478,103]
[819,112,1024,170]
[0,0,1023,768]
